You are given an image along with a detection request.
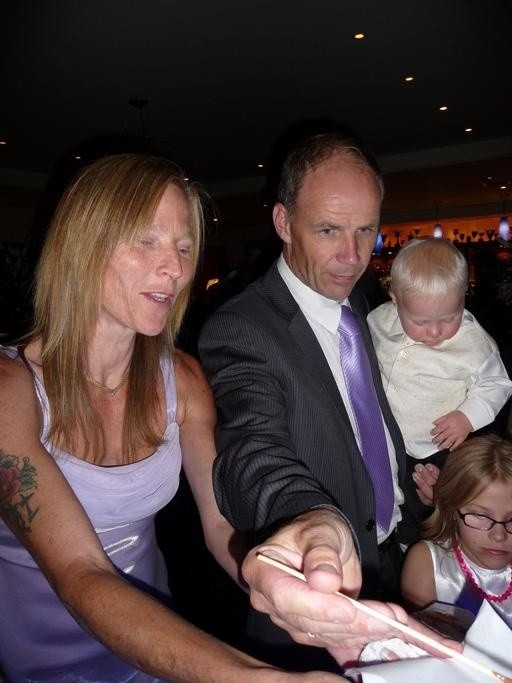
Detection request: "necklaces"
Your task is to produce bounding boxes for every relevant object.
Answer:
[452,536,512,603]
[85,372,129,396]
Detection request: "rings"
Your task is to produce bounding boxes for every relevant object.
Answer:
[307,632,315,638]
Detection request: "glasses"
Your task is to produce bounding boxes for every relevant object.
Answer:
[456,507,512,535]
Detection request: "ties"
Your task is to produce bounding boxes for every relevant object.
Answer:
[338,305,394,534]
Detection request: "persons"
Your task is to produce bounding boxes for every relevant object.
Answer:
[198,133,441,650]
[366,235,512,546]
[0,152,465,683]
[401,434,512,632]
